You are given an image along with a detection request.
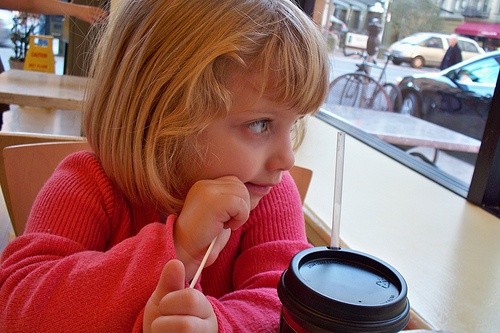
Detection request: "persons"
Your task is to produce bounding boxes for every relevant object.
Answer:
[1,1,331,332]
[440,41,463,71]
[363,18,382,68]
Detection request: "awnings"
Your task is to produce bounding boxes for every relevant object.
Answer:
[455,21,500,39]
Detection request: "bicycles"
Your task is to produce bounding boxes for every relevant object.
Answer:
[339,44,403,118]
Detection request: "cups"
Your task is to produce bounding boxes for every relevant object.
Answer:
[277,245,410,333]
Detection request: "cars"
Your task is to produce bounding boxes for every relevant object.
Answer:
[388,48,500,140]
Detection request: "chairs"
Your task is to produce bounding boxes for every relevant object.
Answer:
[1,132,313,238]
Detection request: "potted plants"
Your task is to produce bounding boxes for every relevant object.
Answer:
[8,10,42,69]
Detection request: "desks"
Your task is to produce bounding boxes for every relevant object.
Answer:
[317,103,482,164]
[0,68,95,110]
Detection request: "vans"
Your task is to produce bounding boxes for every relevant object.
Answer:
[387,32,487,69]
